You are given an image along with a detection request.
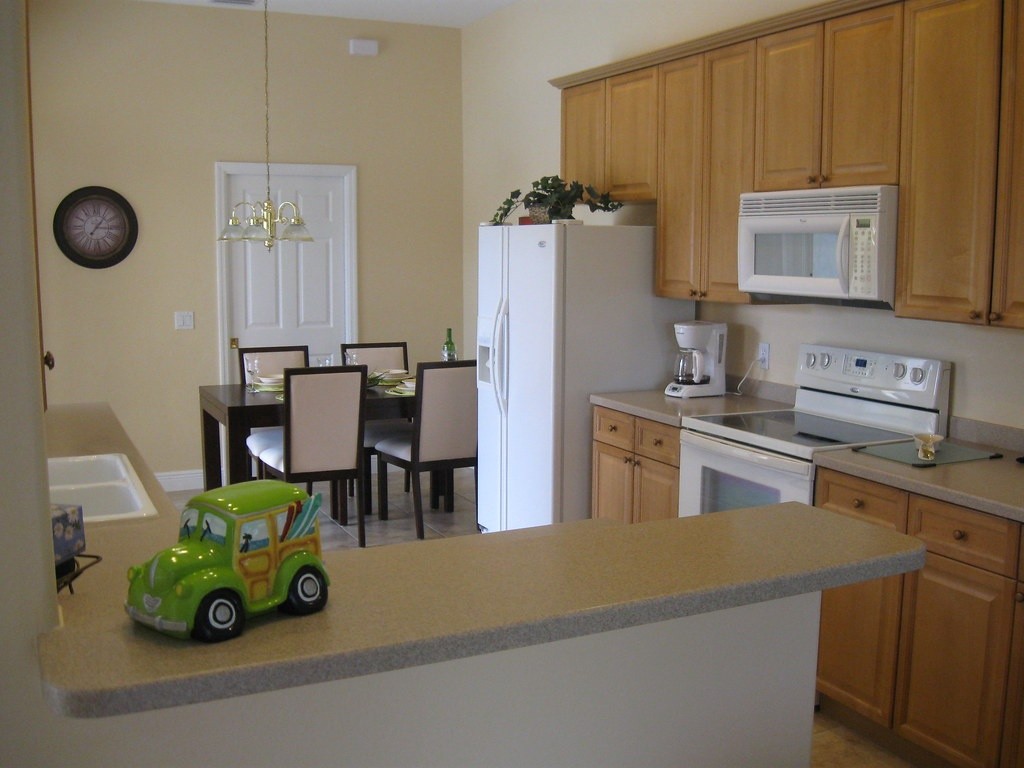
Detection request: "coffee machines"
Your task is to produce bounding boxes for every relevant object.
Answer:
[664,320,728,399]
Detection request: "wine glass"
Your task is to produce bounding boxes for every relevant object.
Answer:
[246,357,261,393]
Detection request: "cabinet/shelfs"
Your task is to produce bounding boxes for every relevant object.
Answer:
[547,0,1024,330]
[591,405,1024,768]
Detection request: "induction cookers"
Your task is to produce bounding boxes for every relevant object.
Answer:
[680,343,951,462]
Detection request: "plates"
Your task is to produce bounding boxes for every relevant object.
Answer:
[254,382,283,387]
[378,376,412,381]
[395,386,415,391]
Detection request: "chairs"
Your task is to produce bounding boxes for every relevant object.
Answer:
[238,340,477,547]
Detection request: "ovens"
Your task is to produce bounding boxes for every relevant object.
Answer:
[678,428,815,518]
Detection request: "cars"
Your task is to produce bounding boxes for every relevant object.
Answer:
[123,478,329,645]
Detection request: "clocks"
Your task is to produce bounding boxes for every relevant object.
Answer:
[52,185,139,271]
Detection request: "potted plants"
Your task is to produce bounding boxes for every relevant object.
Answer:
[489,174,625,226]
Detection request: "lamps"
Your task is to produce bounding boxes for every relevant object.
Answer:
[216,0,316,256]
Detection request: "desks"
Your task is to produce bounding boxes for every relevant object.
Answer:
[199,375,457,514]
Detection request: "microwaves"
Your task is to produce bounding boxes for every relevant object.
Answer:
[737,184,899,312]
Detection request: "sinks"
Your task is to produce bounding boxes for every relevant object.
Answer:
[50,484,146,519]
[47,457,127,485]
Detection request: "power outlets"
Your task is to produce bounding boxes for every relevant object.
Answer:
[759,343,770,370]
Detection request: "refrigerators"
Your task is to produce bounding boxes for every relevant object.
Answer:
[476,221,695,534]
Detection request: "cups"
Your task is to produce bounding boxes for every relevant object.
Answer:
[344,352,360,366]
[317,355,332,367]
[445,351,458,361]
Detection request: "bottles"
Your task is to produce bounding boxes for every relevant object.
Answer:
[443,327,454,361]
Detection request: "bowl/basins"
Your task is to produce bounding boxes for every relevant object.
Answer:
[402,380,416,388]
[257,375,283,383]
[373,368,407,378]
